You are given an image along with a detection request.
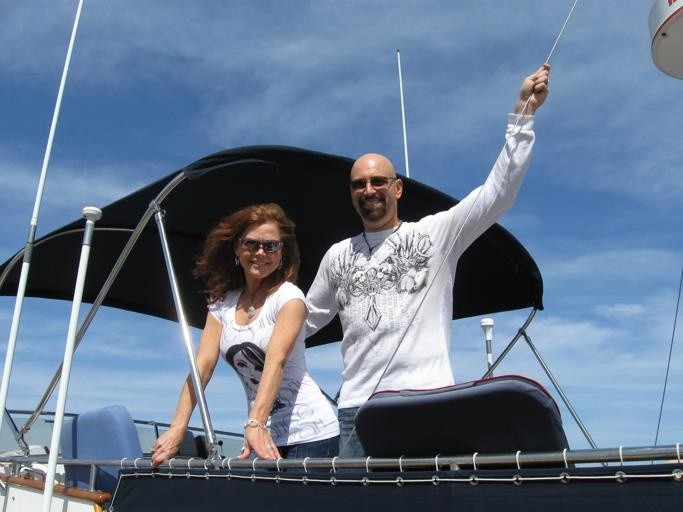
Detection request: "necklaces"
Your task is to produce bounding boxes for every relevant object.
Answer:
[246,285,267,320]
[362,220,402,261]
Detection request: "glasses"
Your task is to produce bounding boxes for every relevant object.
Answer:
[240,236,285,254]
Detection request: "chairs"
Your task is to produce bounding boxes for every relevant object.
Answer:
[60,405,145,511]
[354,374,575,469]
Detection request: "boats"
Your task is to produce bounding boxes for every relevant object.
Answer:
[0,2,682,512]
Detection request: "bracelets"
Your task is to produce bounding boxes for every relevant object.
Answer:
[242,419,269,432]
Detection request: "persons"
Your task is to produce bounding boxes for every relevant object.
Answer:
[302,61,552,475]
[149,202,341,474]
